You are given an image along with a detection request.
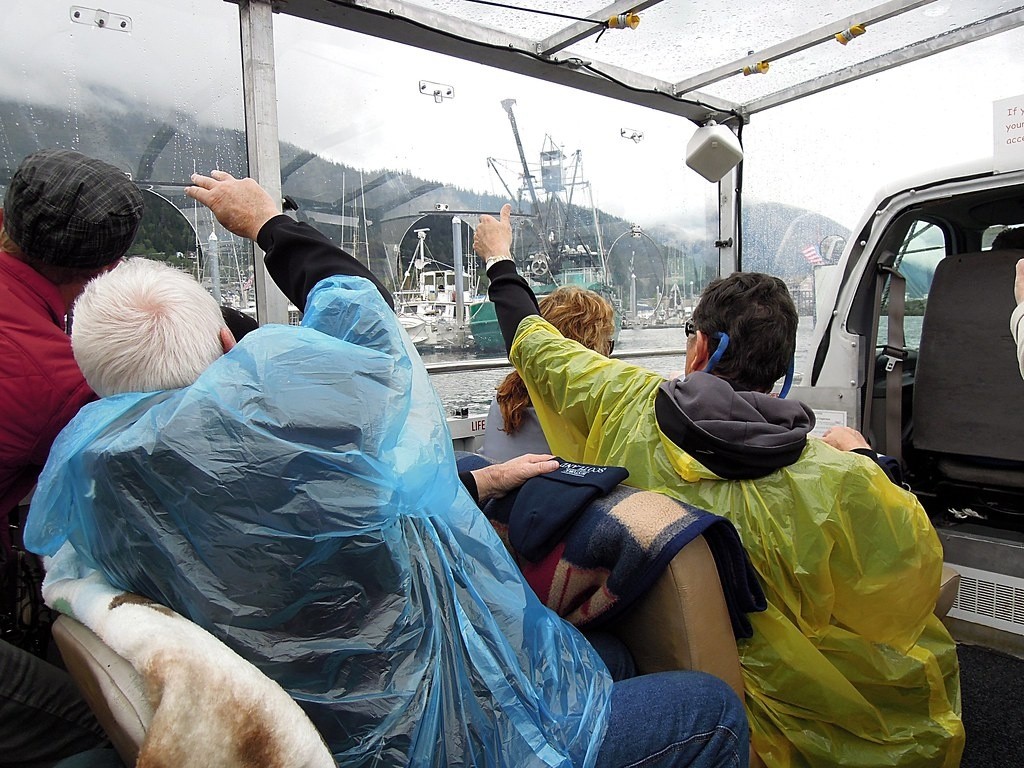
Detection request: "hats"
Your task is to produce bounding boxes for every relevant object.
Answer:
[3,148,143,270]
[508,457,629,563]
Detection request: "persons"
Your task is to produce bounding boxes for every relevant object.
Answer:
[68,163,750,767]
[484,274,616,467]
[1009,258,1024,377]
[0,146,144,768]
[470,203,966,768]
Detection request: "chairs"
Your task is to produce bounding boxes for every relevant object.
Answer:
[480,479,747,709]
[912,248,1024,462]
[48,584,337,768]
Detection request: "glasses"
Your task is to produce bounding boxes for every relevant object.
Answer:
[607,339,614,355]
[685,318,709,337]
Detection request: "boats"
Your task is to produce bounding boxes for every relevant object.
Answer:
[387,227,473,350]
[467,148,624,355]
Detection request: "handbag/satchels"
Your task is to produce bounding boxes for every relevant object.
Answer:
[0,506,62,646]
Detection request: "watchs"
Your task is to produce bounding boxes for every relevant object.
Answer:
[484,255,515,274]
